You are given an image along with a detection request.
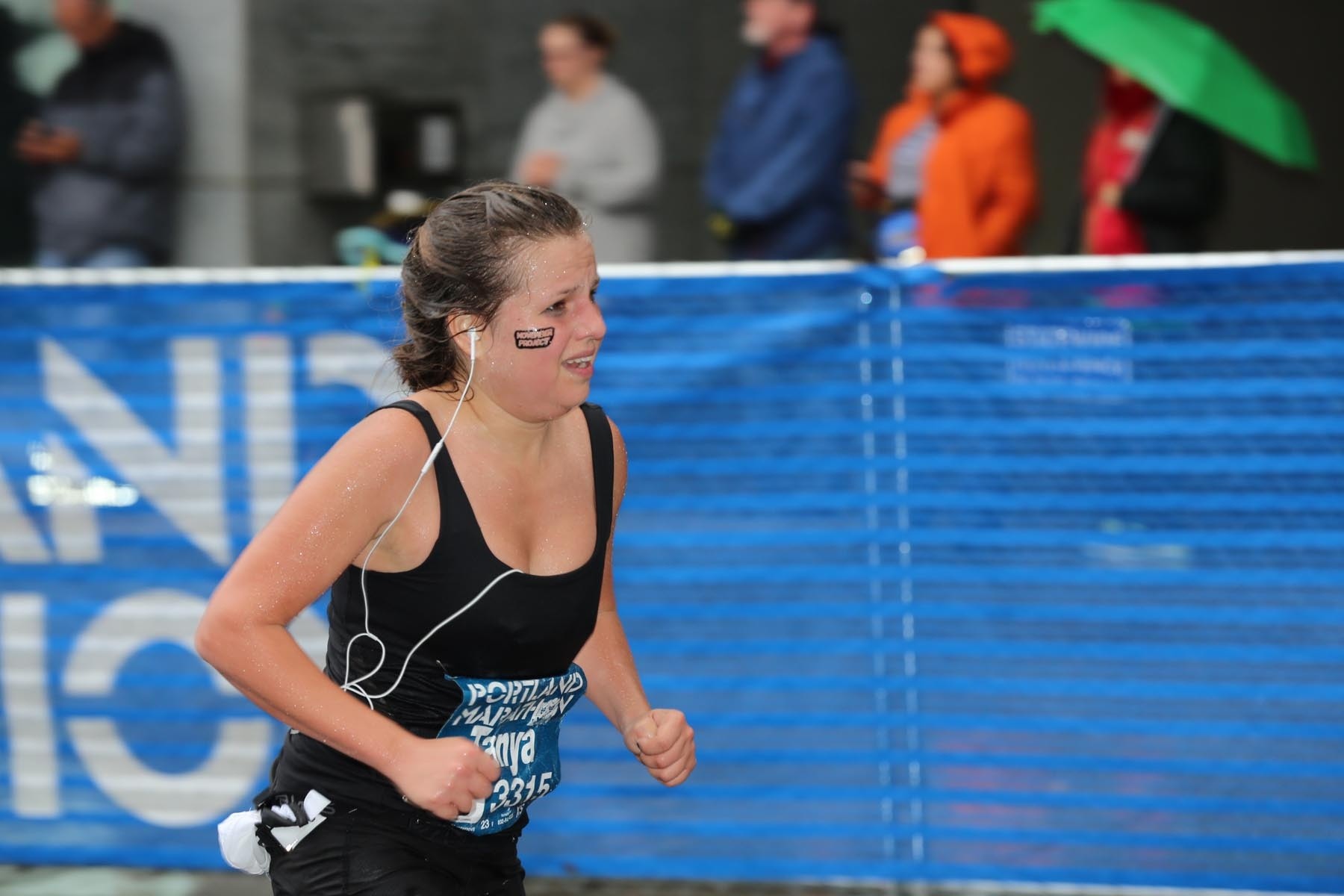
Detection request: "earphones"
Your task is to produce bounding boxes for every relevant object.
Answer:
[466,326,480,359]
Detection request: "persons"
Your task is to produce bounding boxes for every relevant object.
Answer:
[196,179,695,896]
[10,0,189,272]
[509,10,664,267]
[701,0,860,261]
[1063,62,1230,260]
[847,9,1041,258]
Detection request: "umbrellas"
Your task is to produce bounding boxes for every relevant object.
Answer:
[1027,0,1325,177]
